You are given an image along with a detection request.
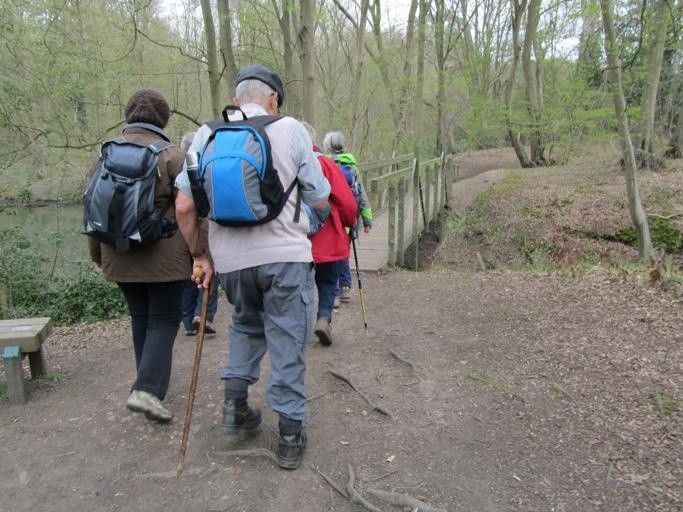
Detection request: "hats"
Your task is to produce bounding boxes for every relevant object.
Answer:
[324,132,347,154]
[124,89,169,129]
[235,64,284,108]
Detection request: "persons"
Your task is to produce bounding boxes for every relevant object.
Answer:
[171,132,226,336]
[322,132,374,307]
[85,91,208,423]
[173,63,331,471]
[296,120,359,349]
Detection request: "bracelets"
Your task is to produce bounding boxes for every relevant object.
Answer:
[187,249,209,259]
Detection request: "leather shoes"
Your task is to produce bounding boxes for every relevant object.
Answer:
[278,427,307,469]
[221,398,261,430]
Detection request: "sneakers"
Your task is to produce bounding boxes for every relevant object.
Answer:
[339,286,350,299]
[192,315,216,333]
[332,295,340,307]
[185,329,198,336]
[125,389,174,422]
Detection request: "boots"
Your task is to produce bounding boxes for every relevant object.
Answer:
[314,316,333,345]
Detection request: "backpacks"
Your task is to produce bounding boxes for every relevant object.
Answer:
[340,162,364,217]
[80,136,178,253]
[183,105,306,227]
[302,151,332,237]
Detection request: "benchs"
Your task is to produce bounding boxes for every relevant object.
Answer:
[0,317,53,405]
